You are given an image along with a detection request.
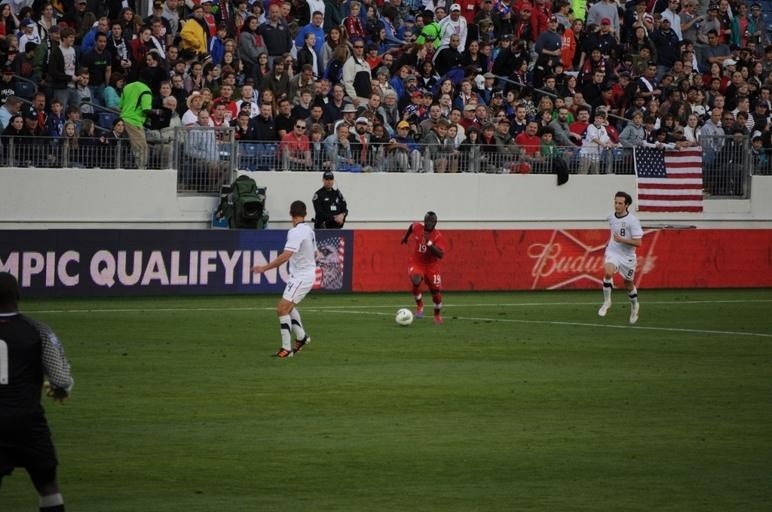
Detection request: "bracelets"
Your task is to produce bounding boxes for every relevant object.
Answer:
[426,240,432,246]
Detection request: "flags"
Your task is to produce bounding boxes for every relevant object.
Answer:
[633,146,704,213]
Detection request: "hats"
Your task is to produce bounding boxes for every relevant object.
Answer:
[707,3,720,14]
[341,104,357,113]
[547,16,558,23]
[450,4,461,11]
[397,121,410,130]
[601,18,610,25]
[186,91,203,109]
[723,59,737,68]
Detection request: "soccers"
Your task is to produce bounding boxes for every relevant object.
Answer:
[395,308,412,326]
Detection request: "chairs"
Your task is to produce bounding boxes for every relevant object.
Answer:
[112,112,119,123]
[181,142,280,182]
[96,112,115,131]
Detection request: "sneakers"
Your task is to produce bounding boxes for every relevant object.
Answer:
[416,300,424,318]
[598,301,612,316]
[433,312,442,324]
[271,347,293,358]
[630,303,640,323]
[293,335,311,354]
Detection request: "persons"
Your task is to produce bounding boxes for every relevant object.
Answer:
[401,211,445,325]
[0,0,772,176]
[249,200,317,358]
[597,192,644,323]
[1,270,74,512]
[312,171,348,228]
